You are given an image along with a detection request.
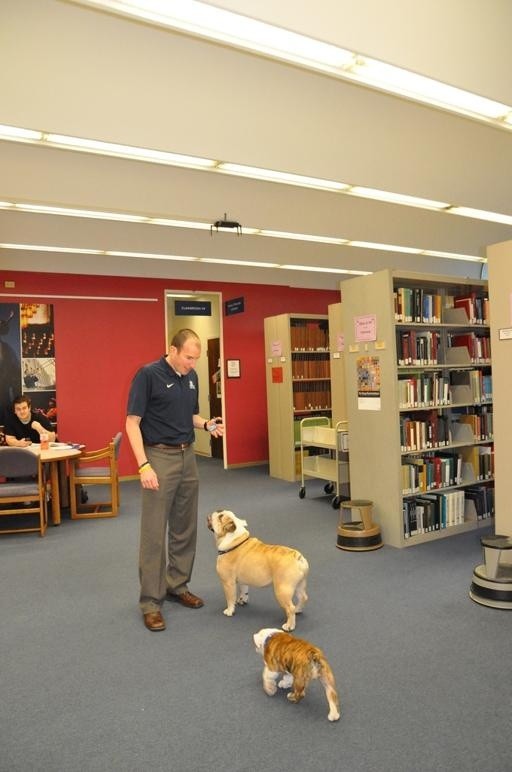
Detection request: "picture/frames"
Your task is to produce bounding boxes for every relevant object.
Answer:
[226,358,241,378]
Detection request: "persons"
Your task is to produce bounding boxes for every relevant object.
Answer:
[4,393,93,510]
[123,326,224,634]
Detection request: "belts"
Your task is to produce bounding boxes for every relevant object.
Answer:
[153,442,189,449]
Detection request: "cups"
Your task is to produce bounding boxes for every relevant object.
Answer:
[40,433,49,451]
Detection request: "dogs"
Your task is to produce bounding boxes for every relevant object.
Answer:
[252,627,342,723]
[206,509,310,632]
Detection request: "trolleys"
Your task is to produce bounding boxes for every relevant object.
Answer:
[300,416,350,509]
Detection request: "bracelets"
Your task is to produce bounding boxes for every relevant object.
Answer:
[204,420,208,431]
[139,465,152,474]
[138,461,150,469]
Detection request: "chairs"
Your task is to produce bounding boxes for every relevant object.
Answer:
[0,431,123,538]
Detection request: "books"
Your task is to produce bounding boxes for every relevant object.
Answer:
[393,287,494,537]
[291,327,333,474]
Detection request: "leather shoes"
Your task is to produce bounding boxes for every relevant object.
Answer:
[165,591,203,608]
[144,610,166,631]
